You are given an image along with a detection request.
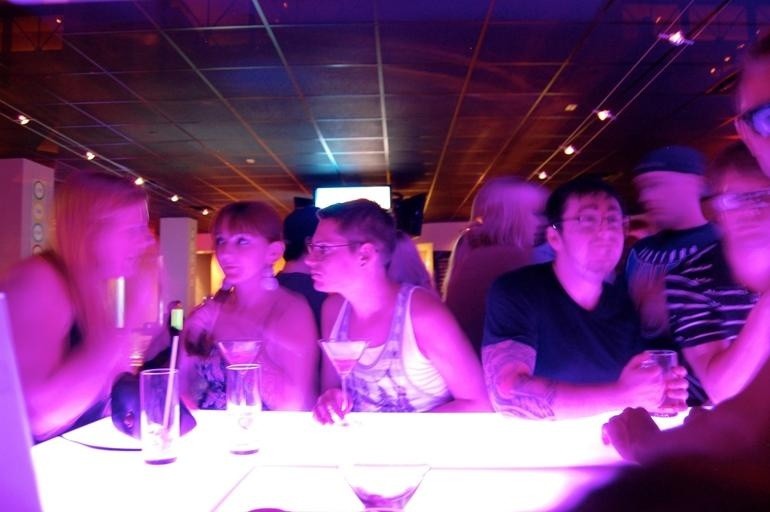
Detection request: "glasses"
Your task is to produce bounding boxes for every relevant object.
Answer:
[559,209,631,229]
[739,99,770,139]
[307,242,380,258]
[702,188,770,213]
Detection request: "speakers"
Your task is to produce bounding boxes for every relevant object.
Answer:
[0,157,55,260]
[161,217,197,313]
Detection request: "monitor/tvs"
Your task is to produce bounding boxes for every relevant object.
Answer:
[294,197,312,212]
[398,193,423,236]
[312,185,392,219]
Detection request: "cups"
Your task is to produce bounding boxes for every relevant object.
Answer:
[348,451,426,512]
[224,362,264,457]
[638,349,681,418]
[139,367,187,471]
[217,338,266,374]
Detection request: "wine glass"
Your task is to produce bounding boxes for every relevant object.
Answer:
[318,337,369,433]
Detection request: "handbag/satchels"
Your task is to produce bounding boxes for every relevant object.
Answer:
[110,372,196,440]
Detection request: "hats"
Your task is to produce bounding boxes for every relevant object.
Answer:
[632,147,704,177]
[283,206,323,260]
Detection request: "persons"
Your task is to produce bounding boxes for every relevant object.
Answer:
[308,199,495,425]
[443,180,547,343]
[623,145,718,308]
[277,208,329,324]
[0,169,165,448]
[664,138,770,409]
[574,353,770,512]
[389,231,432,290]
[480,177,689,418]
[163,196,321,412]
[732,42,770,174]
